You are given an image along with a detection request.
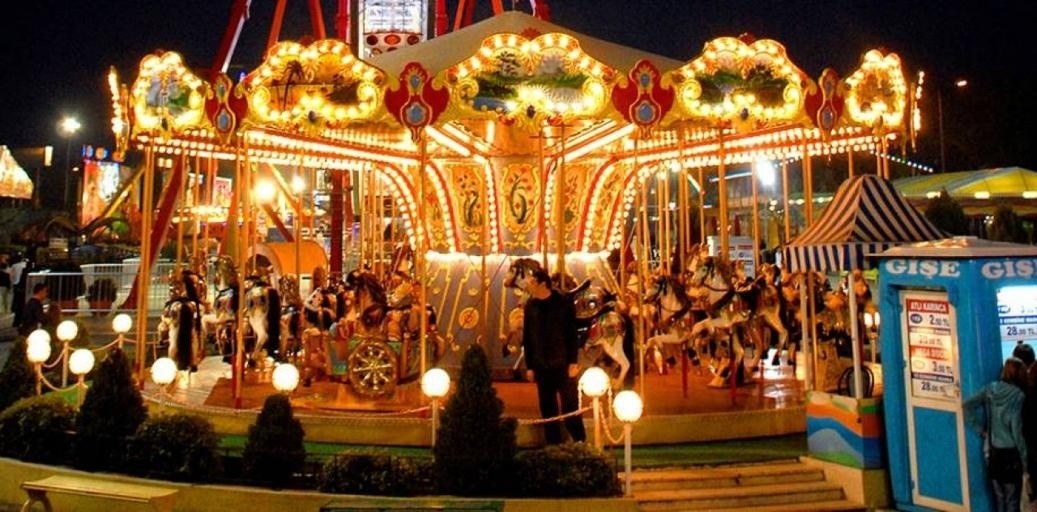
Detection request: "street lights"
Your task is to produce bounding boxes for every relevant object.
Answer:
[937,77,969,175]
[60,115,81,209]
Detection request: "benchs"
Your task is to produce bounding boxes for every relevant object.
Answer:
[316,494,503,511]
[18,475,179,510]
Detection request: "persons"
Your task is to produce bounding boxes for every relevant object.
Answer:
[0,253,62,353]
[962,359,1030,511]
[1021,360,1036,505]
[157,245,878,390]
[1012,343,1035,368]
[523,272,586,444]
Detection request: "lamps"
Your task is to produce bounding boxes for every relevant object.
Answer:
[271,362,299,397]
[421,364,450,452]
[147,357,175,418]
[25,310,136,409]
[578,366,642,500]
